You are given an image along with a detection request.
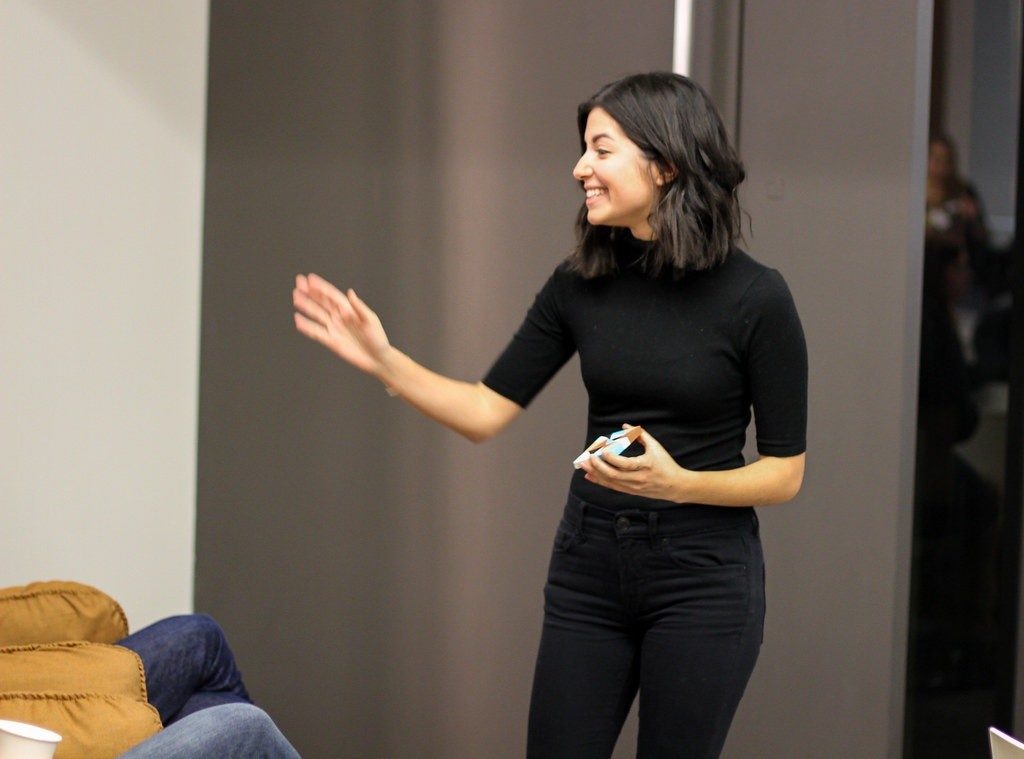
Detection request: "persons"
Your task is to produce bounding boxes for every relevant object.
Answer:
[901,133,1024,759]
[111,614,302,759]
[293,72,808,759]
